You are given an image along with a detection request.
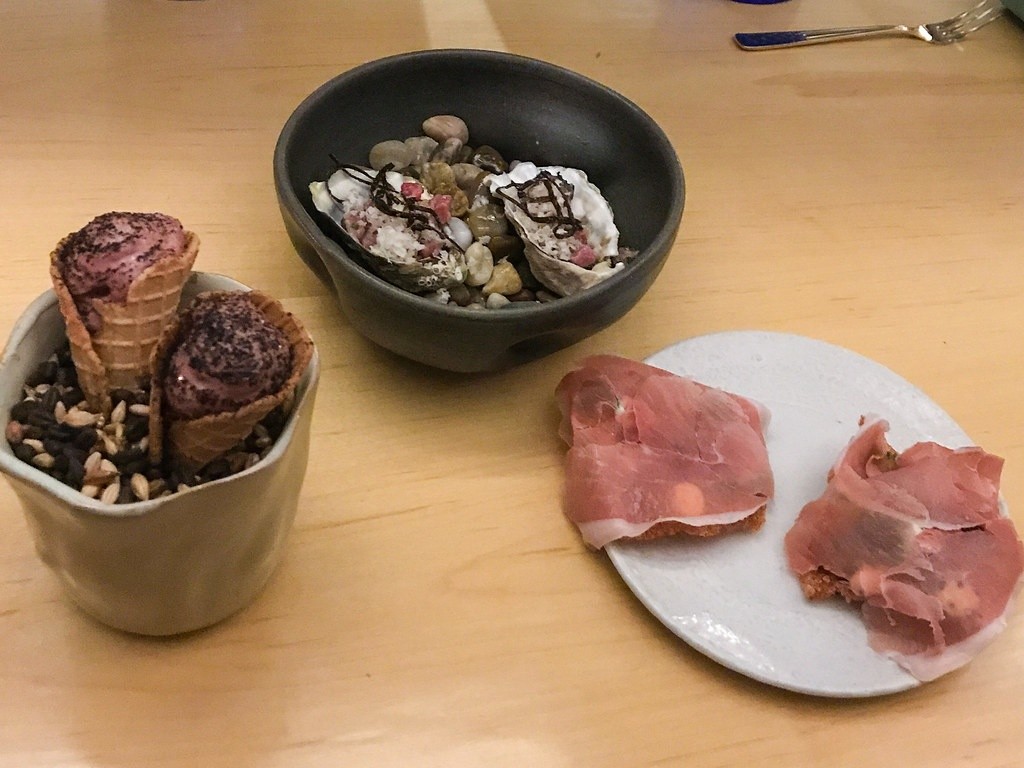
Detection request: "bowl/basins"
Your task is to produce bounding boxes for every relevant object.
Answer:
[271,48,690,376]
[0,267,321,638]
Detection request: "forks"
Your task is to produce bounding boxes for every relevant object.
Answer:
[731,0,1005,53]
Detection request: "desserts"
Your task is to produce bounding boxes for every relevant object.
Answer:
[40,199,316,467]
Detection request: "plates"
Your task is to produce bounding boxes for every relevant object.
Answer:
[599,325,1014,699]
